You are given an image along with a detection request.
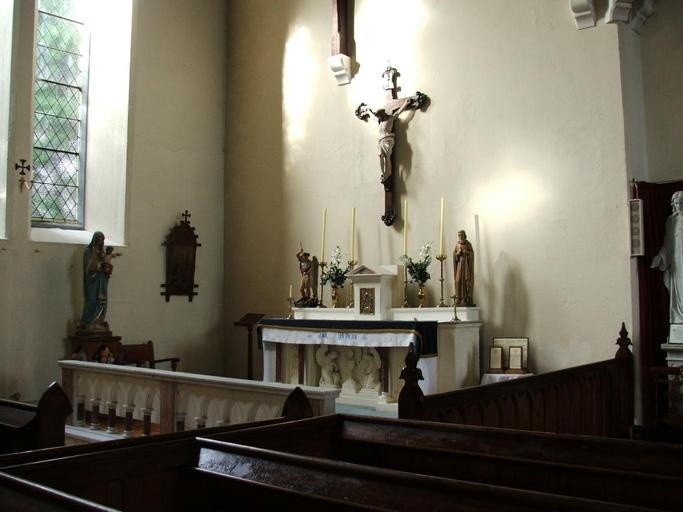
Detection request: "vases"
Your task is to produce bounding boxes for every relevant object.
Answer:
[418,285,425,305]
[330,287,339,307]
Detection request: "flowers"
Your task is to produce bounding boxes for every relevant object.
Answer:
[320,246,357,288]
[400,241,433,285]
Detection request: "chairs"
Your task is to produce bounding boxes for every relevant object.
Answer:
[118,340,181,373]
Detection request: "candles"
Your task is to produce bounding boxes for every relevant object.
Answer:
[403,200,408,256]
[321,208,327,262]
[452,280,456,296]
[289,284,293,297]
[352,207,355,261]
[439,197,446,256]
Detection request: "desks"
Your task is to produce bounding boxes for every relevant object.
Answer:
[480,373,534,386]
[68,338,121,363]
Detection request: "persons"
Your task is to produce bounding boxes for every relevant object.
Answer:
[95,246,123,275]
[79,230,113,331]
[296,248,312,298]
[365,100,412,181]
[452,230,475,306]
[650,190,683,343]
